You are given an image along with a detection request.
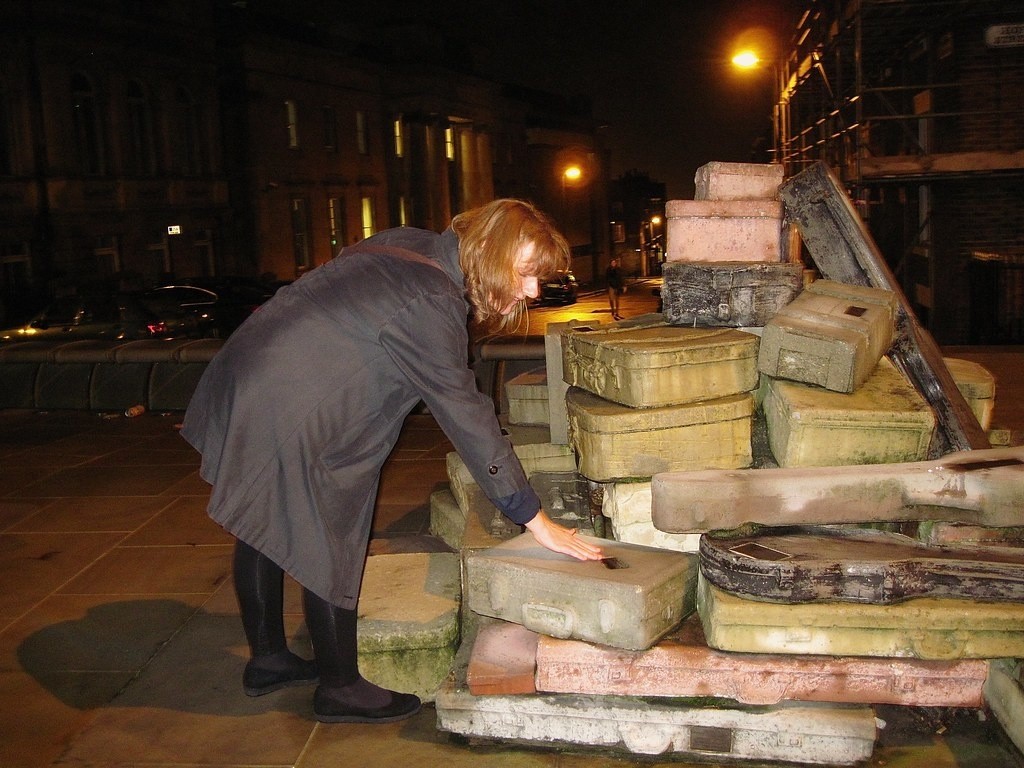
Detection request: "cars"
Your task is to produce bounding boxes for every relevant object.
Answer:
[541,271,578,304]
[0,269,282,347]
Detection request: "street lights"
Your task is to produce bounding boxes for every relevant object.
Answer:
[561,167,582,210]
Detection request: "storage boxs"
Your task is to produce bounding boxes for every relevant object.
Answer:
[428,162,996,549]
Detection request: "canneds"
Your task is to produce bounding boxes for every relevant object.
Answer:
[125,405,144,417]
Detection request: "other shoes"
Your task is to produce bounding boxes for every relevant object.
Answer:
[243,654,319,697]
[313,675,421,724]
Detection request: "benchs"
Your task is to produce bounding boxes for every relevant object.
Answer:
[358,554,461,651]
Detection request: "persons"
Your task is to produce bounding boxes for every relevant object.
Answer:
[604,259,627,316]
[178,198,604,723]
